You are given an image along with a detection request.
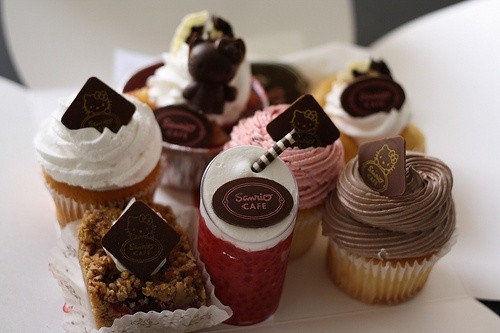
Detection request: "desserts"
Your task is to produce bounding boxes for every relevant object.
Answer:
[34,10,457,329]
